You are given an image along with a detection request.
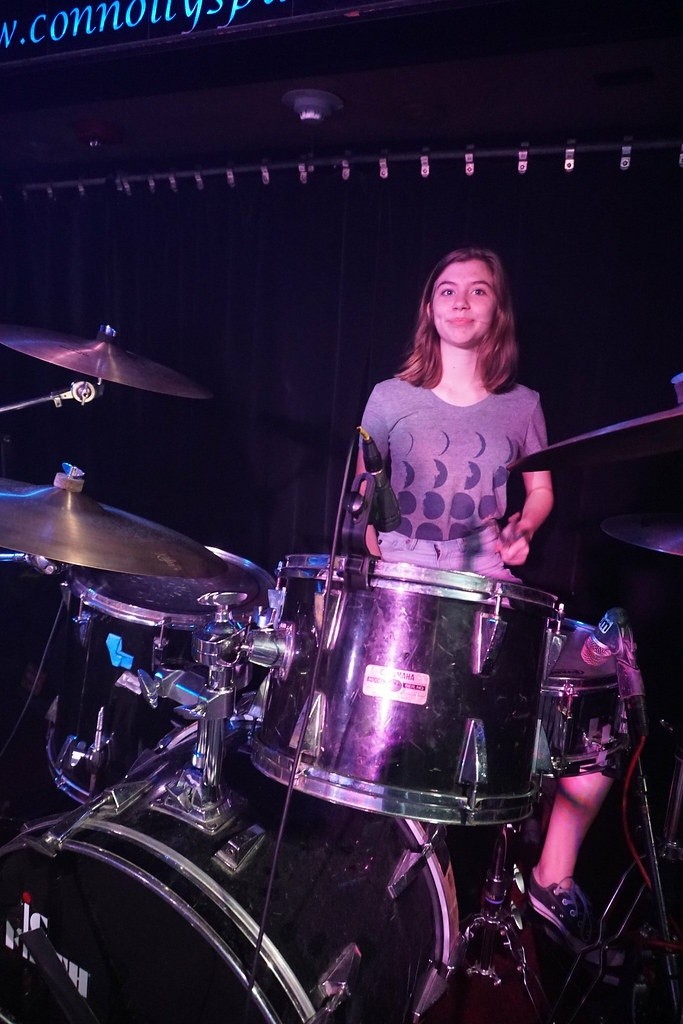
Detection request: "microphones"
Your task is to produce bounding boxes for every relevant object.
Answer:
[581,608,626,667]
[362,436,402,533]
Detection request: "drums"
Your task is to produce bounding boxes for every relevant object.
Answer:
[0,712,460,1024]
[536,614,631,780]
[242,551,560,825]
[43,545,274,811]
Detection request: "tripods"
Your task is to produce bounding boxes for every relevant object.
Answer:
[414,624,683,1024]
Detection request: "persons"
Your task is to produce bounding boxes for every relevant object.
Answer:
[355,248,625,966]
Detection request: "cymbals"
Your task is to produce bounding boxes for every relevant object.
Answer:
[0,325,216,402]
[505,402,683,473]
[602,504,683,558]
[0,478,227,577]
